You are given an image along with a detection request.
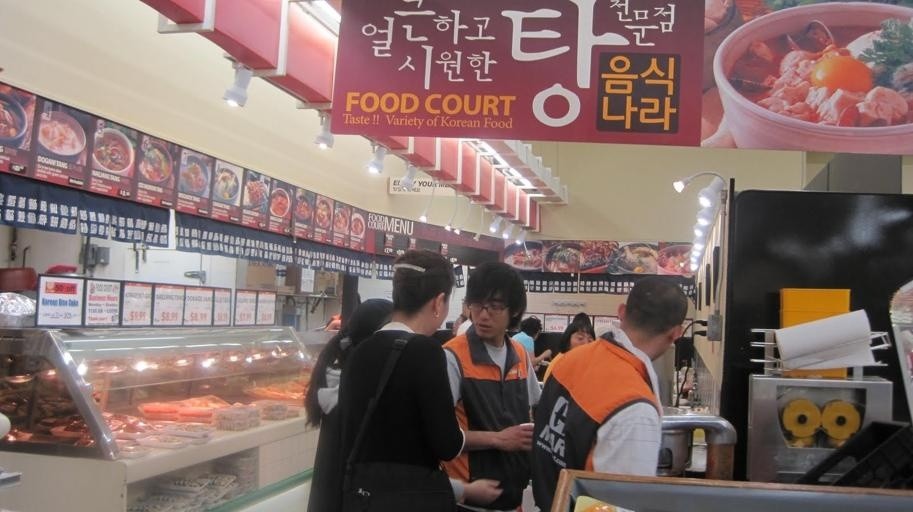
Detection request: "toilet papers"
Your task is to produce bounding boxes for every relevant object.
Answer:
[774,308,888,373]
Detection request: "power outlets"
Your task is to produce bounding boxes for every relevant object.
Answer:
[706,313,722,341]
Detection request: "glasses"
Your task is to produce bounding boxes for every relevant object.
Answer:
[467,302,508,315]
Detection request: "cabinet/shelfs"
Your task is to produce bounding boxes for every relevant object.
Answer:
[0,324,318,512]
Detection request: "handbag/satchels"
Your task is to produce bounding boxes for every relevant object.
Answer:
[345,461,457,511]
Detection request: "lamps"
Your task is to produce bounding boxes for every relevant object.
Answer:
[363,146,389,173]
[223,68,252,109]
[516,229,528,244]
[502,223,516,240]
[488,216,503,232]
[686,240,707,273]
[672,171,729,196]
[697,205,718,226]
[444,214,456,232]
[399,168,418,192]
[454,226,464,235]
[473,228,481,243]
[698,174,724,210]
[311,112,338,149]
[419,204,430,223]
[694,225,713,237]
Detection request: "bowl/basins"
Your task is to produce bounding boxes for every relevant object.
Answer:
[179,155,207,196]
[333,207,349,232]
[612,242,657,274]
[657,244,693,275]
[269,188,290,217]
[294,194,312,223]
[212,167,240,202]
[315,199,331,228]
[135,141,172,184]
[542,242,579,273]
[351,212,365,239]
[710,1,913,152]
[37,110,86,164]
[502,241,542,271]
[91,127,134,174]
[0,92,28,147]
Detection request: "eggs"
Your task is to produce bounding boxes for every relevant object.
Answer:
[812,55,873,93]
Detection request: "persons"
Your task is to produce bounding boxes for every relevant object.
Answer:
[444,261,543,511]
[532,275,689,511]
[511,316,553,372]
[304,299,506,511]
[543,319,596,392]
[338,249,463,512]
